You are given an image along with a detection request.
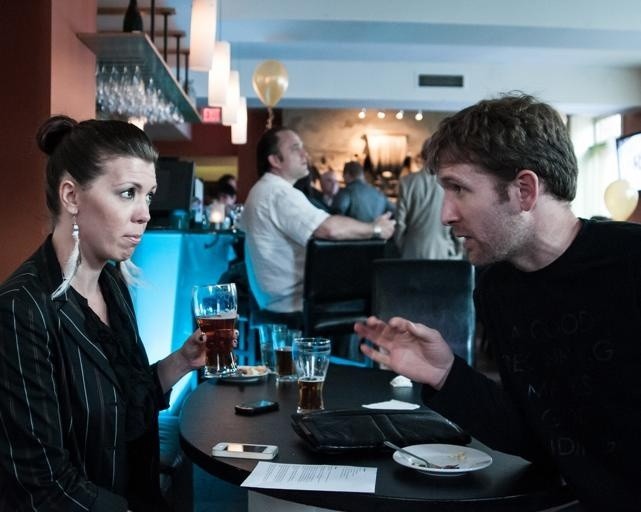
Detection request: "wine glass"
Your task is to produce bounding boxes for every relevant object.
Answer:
[93,62,185,127]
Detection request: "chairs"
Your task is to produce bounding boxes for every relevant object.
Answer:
[370,257,478,371]
[303,237,390,369]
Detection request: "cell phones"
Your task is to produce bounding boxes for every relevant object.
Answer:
[235,399,279,417]
[212,442,279,461]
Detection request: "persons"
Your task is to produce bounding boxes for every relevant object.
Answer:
[394,138,465,259]
[0,113,239,511]
[320,170,339,212]
[296,165,329,213]
[244,127,394,330]
[217,174,238,190]
[206,183,250,316]
[353,89,639,511]
[330,160,396,222]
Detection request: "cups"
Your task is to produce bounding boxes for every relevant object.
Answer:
[209,201,245,231]
[258,321,333,414]
[192,283,242,379]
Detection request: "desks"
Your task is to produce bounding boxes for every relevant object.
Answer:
[176,359,588,511]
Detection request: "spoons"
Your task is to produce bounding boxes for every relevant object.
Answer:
[383,440,460,470]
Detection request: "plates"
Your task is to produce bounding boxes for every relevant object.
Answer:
[394,443,495,476]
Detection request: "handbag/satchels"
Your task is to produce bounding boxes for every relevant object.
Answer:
[290,407,471,452]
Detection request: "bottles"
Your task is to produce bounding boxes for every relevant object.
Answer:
[168,194,208,231]
[122,0,145,33]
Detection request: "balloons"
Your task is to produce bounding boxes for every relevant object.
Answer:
[253,62,288,111]
[604,181,638,222]
